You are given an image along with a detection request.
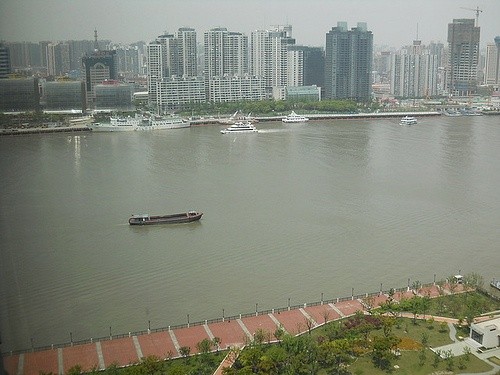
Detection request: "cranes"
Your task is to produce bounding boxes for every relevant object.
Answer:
[461,4,483,27]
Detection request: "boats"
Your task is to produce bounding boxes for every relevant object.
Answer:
[282,115,309,124]
[152,120,191,129]
[221,122,259,135]
[90,119,138,132]
[129,213,203,225]
[400,116,418,127]
